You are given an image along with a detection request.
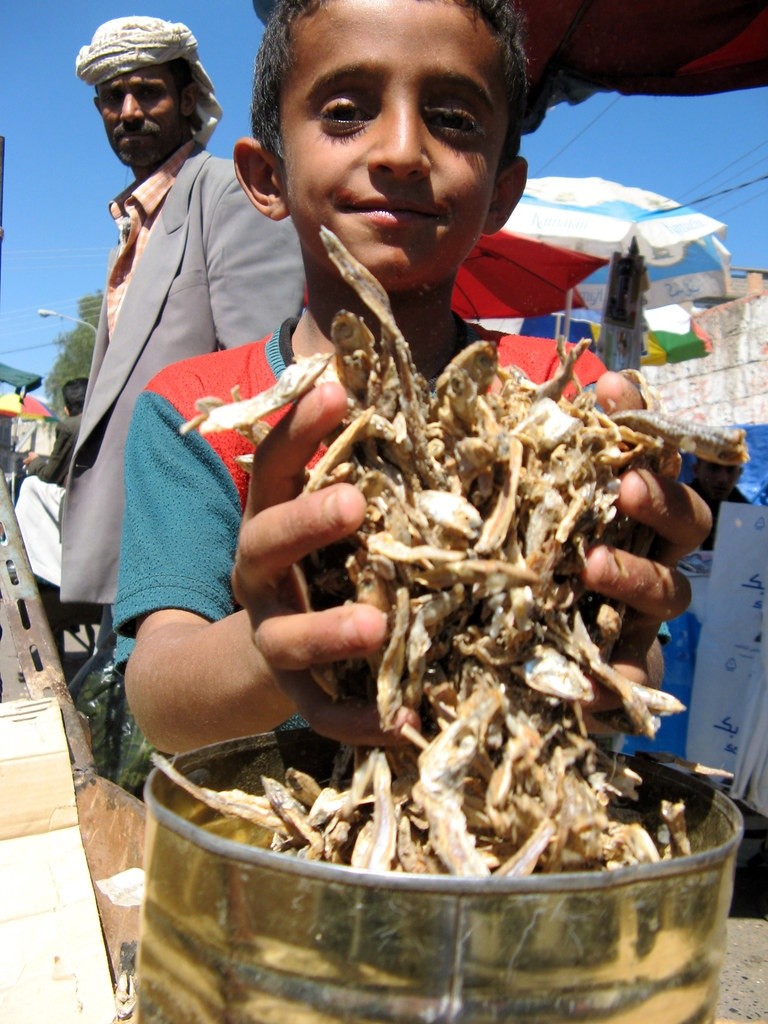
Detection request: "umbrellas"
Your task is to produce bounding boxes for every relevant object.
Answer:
[519,305,713,367]
[514,0,768,134]
[449,230,608,322]
[0,393,57,453]
[0,364,43,395]
[501,175,734,347]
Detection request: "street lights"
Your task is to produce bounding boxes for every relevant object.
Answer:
[38,309,97,336]
[11,418,45,506]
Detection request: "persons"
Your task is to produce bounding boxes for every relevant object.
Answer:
[106,0,712,756]
[58,14,307,602]
[6,456,27,505]
[685,455,753,551]
[13,376,89,591]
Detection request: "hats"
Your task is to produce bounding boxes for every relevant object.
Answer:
[75,15,223,146]
[694,429,750,464]
